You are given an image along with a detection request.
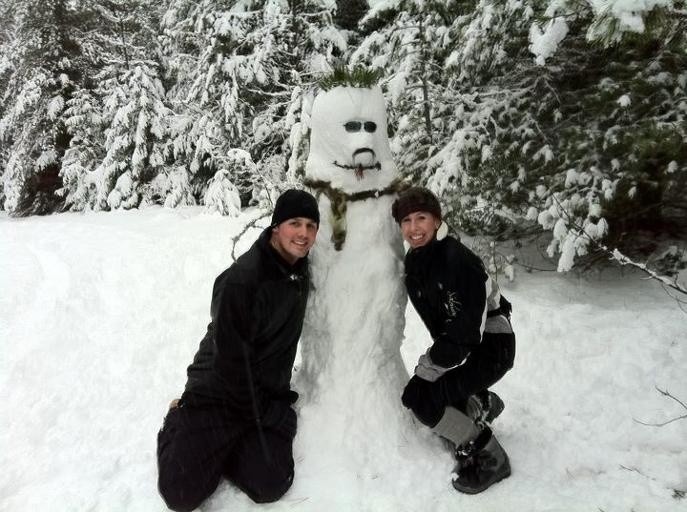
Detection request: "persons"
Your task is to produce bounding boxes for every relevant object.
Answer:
[392,187,515,494]
[157,188,319,512]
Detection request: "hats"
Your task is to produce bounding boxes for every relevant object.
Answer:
[271,190,319,230]
[392,187,441,227]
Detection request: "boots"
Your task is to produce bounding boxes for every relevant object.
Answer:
[464,389,505,427]
[452,426,511,494]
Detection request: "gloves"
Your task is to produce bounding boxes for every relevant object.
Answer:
[413,348,450,381]
